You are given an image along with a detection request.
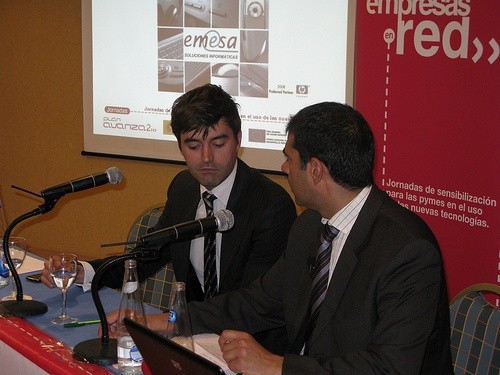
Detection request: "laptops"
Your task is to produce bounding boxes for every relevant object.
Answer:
[122,317,241,375]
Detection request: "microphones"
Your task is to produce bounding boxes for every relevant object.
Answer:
[9,166,123,199]
[101,210,235,249]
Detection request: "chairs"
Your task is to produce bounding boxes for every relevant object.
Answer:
[124,203,178,311]
[449,283,500,375]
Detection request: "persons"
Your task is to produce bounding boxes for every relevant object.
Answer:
[41,83,298,358]
[95,103,453,374]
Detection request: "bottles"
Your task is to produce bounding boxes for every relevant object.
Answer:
[166,282,194,370]
[0,202,9,289]
[117,259,147,375]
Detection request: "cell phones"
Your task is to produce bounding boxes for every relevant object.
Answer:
[24,272,57,282]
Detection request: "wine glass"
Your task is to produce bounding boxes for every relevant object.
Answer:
[0,237,32,301]
[49,253,79,325]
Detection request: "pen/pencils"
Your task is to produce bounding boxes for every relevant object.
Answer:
[64,320,101,328]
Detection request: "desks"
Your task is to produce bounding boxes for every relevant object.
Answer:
[0,240,165,375]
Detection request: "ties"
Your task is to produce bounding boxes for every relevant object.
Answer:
[202,192,217,298]
[306,224,339,346]
